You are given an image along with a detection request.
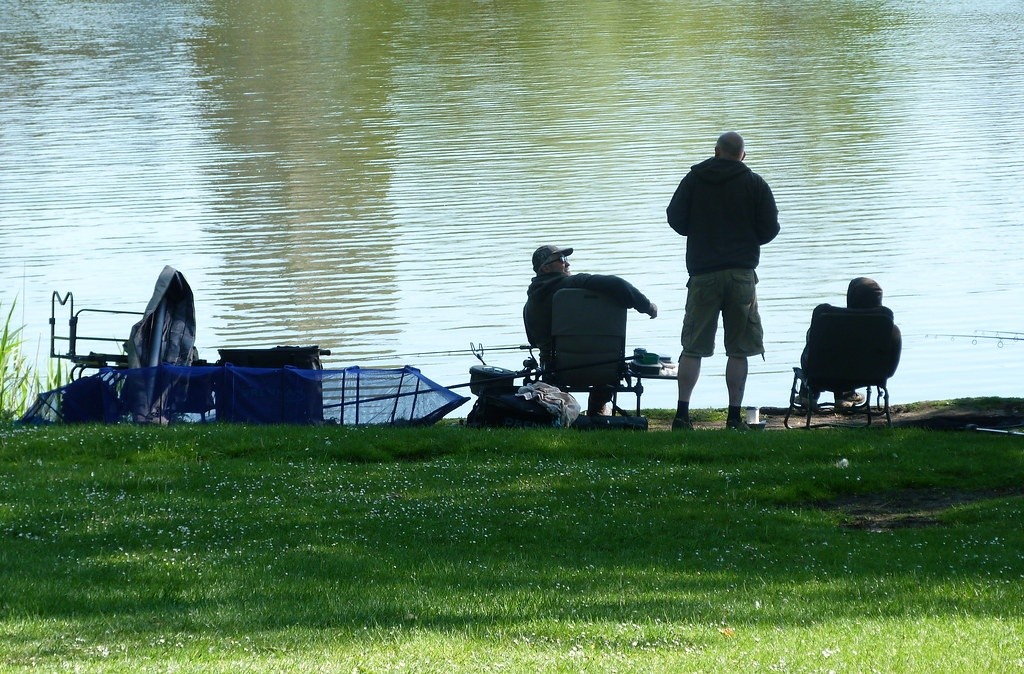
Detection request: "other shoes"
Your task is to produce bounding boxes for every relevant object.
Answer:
[726,418,753,431]
[841,392,865,407]
[671,418,694,431]
[793,395,817,407]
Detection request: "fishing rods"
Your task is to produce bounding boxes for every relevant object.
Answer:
[359,345,533,360]
[323,355,641,411]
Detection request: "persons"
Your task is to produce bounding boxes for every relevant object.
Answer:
[665,131,781,429]
[522,245,657,413]
[798,277,901,413]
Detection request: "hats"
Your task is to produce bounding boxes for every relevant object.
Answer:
[532,244,573,273]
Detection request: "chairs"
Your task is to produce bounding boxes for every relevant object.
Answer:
[48,291,208,427]
[783,302,895,433]
[538,288,629,429]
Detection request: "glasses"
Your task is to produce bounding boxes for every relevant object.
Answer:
[544,255,567,266]
